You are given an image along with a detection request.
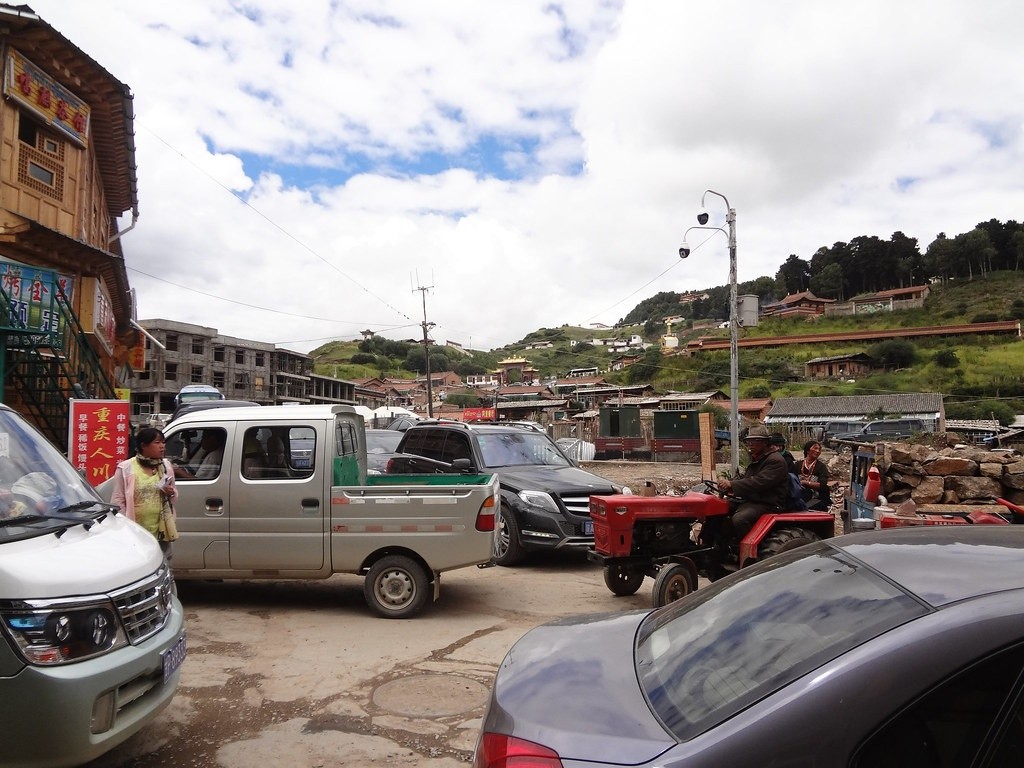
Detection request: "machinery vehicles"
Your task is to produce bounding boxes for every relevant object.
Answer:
[587,451,1024,609]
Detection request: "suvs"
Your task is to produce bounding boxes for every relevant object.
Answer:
[830,419,924,454]
[387,420,632,566]
[818,421,869,448]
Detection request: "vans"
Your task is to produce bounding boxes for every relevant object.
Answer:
[0,405,186,768]
[165,400,269,456]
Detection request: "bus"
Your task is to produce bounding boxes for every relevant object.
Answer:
[173,385,225,408]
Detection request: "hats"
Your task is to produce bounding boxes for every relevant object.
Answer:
[769,432,786,444]
[745,424,772,439]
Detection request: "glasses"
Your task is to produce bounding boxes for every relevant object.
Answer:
[746,438,765,443]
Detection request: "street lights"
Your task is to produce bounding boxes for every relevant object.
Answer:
[678,190,739,478]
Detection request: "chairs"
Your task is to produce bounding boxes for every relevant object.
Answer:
[245,438,262,477]
[787,472,805,510]
[268,437,288,468]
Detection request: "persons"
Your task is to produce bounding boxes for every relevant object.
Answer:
[173,425,224,480]
[770,432,795,473]
[793,441,831,511]
[111,429,180,598]
[716,423,792,542]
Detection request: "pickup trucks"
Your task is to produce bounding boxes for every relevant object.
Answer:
[96,403,501,619]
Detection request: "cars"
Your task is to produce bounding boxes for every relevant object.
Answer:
[473,525,1024,768]
[290,415,427,474]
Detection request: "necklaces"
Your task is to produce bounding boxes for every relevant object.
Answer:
[800,460,816,481]
